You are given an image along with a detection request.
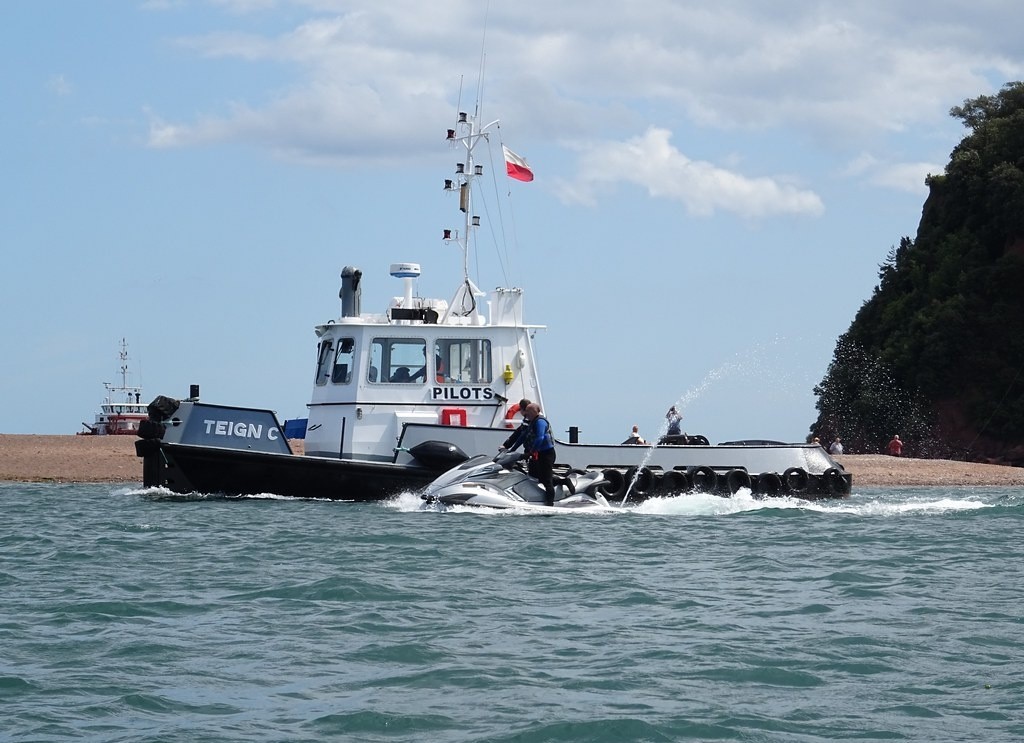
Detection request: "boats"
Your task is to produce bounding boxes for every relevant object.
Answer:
[130,48,855,513]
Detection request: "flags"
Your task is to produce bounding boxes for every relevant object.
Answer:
[502,143,534,183]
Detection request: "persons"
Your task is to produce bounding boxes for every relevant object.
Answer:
[498,398,533,478]
[408,346,443,383]
[347,359,377,382]
[811,437,819,444]
[666,406,682,436]
[628,425,647,444]
[888,435,902,456]
[829,438,843,455]
[522,404,576,506]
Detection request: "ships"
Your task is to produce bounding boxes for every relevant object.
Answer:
[75,337,150,435]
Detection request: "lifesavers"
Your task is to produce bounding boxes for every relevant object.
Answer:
[505,403,522,428]
